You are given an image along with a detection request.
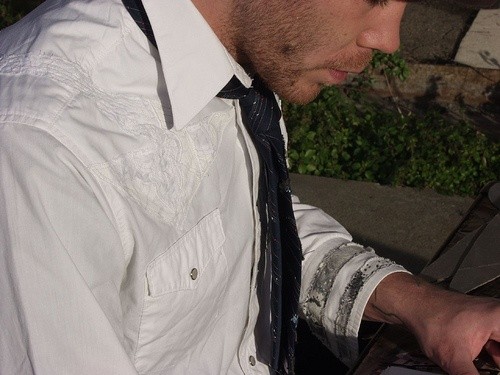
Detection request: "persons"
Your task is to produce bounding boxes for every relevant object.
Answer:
[0,1,500,374]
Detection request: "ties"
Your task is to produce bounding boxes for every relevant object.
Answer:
[121,0,302,375]
